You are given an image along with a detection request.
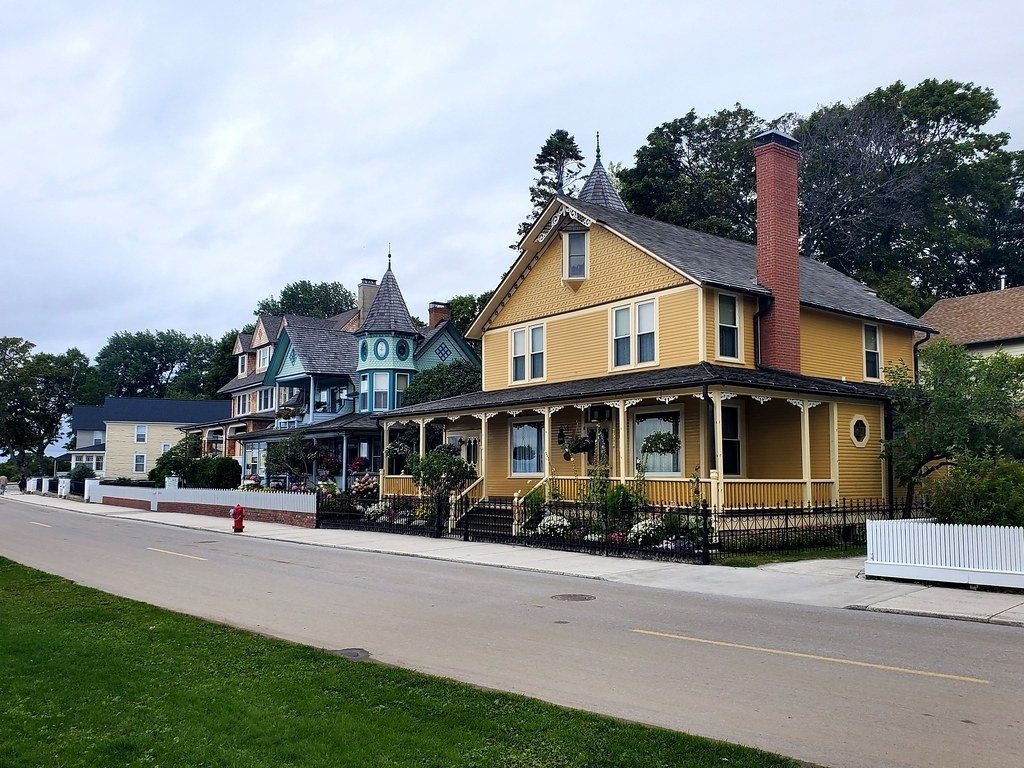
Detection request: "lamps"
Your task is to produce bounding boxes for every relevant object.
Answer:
[458,437,464,447]
[558,424,569,445]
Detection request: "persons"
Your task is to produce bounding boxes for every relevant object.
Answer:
[18,474,26,495]
[0,474,8,495]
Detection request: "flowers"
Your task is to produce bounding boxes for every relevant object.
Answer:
[383,441,412,458]
[322,455,340,472]
[639,432,682,456]
[430,443,461,457]
[347,456,371,471]
[561,435,595,454]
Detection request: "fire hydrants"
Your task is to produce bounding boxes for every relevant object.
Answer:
[230,504,245,533]
